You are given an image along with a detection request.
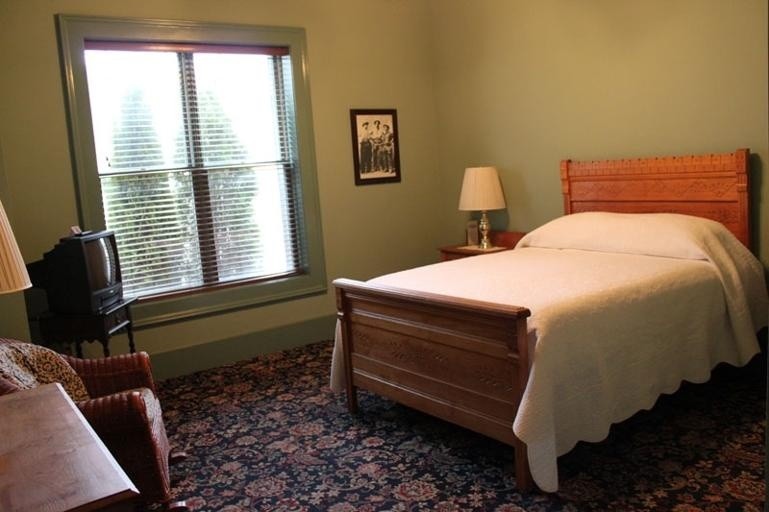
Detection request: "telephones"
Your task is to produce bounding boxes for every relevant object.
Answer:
[465,219,480,247]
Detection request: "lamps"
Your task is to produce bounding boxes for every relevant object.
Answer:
[458,166,507,252]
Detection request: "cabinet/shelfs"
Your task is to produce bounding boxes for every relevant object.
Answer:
[23,260,138,359]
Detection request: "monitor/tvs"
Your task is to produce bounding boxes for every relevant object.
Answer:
[25,229,124,317]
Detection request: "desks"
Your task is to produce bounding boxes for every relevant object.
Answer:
[0,381,141,512]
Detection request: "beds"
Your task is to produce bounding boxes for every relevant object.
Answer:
[331,148,754,494]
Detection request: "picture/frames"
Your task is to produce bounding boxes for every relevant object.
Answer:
[351,109,401,185]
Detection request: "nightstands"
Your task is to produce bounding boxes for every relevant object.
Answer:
[439,230,526,262]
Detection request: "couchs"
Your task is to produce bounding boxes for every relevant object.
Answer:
[0,338,190,512]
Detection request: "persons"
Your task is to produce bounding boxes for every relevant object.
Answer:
[381,124,393,173]
[359,121,372,174]
[370,121,384,172]
